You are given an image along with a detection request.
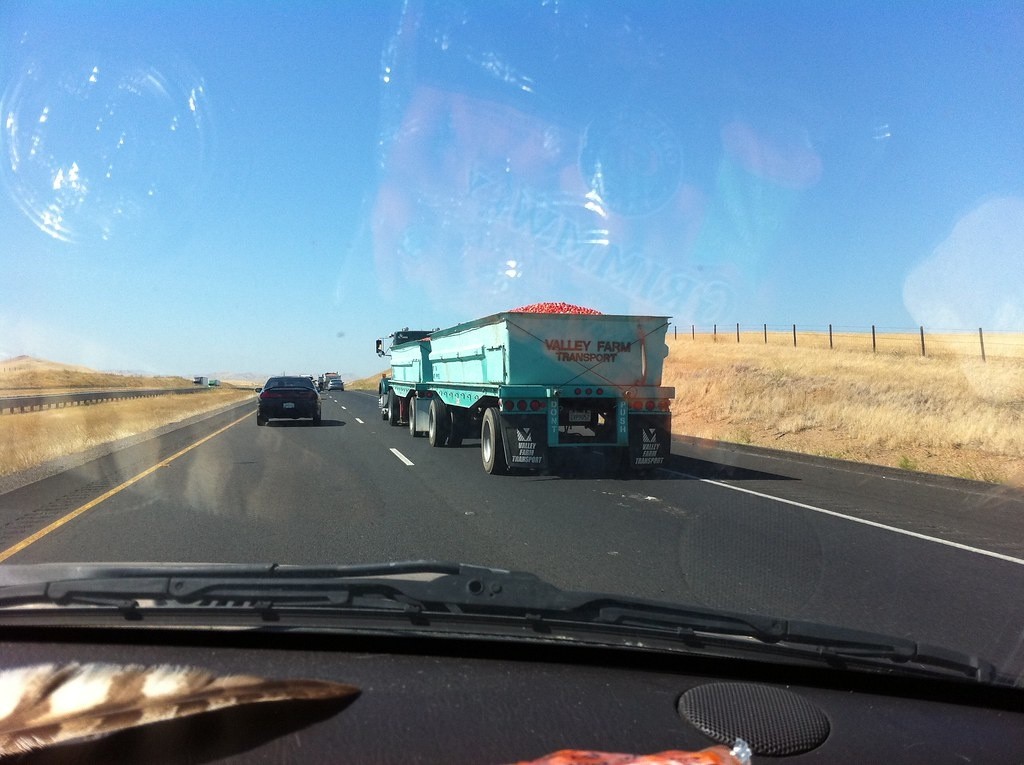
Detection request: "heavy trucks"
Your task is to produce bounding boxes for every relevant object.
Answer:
[376,313,675,476]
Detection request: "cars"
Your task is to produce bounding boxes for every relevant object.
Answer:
[255,376,322,426]
[298,372,344,392]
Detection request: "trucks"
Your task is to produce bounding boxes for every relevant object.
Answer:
[193,377,208,389]
[208,379,220,389]
